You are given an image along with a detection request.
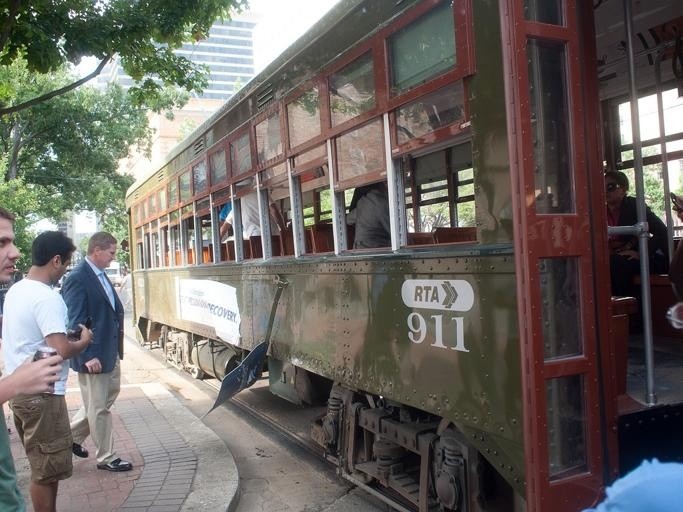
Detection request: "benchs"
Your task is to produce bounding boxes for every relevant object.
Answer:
[611,271,676,394]
[165,226,479,264]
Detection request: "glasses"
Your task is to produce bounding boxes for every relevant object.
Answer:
[607,183,621,192]
[666,303,683,329]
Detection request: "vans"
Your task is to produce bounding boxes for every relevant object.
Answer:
[104,261,122,287]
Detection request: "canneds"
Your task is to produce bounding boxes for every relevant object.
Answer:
[33,347,58,393]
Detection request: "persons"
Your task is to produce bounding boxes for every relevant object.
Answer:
[120,269,133,311]
[219,190,287,258]
[670,196,683,301]
[60,232,133,470]
[2,232,93,512]
[344,183,391,248]
[604,170,667,296]
[0,207,63,512]
[219,202,233,236]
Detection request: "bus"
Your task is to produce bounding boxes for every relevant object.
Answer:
[124,0,683,512]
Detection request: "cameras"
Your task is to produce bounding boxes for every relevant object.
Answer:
[70,316,94,339]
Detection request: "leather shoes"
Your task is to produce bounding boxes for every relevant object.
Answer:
[73,443,89,458]
[97,458,132,471]
